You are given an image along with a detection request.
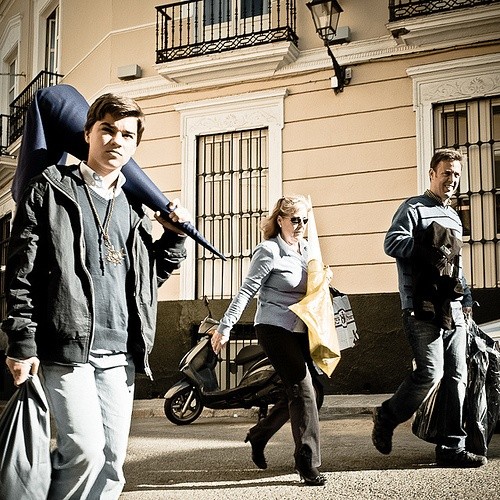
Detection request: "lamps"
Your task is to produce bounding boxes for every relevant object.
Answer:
[306,0,351,96]
[116,64,142,80]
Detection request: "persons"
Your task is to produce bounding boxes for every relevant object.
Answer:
[3,93,186,500]
[211,196,333,486]
[372,146,488,467]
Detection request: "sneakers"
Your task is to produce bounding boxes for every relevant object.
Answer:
[436,446,487,468]
[372,407,393,454]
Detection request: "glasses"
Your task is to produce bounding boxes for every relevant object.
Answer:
[282,216,308,224]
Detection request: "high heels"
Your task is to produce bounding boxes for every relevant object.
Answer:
[244,431,268,470]
[294,466,327,485]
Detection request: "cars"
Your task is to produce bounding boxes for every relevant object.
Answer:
[412,319,500,434]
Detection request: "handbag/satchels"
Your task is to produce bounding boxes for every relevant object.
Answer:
[326,283,361,351]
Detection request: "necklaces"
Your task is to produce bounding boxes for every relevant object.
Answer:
[76,160,127,267]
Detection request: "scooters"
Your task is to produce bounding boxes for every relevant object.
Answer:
[162,295,326,427]
